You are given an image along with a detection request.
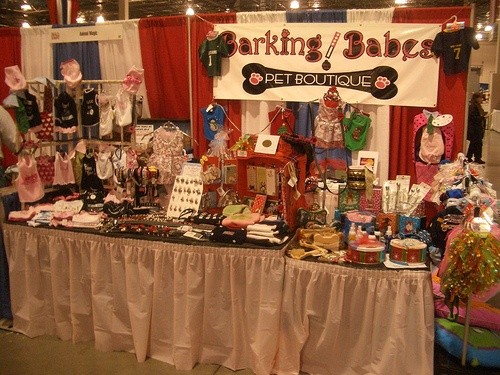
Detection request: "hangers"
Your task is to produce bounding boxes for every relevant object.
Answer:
[207,25,216,39]
[446,15,462,30]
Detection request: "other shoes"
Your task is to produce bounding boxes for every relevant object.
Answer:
[468,159,473,162]
[474,158,485,164]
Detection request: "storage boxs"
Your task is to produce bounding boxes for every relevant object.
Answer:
[375,211,399,238]
[399,215,426,235]
[344,213,376,239]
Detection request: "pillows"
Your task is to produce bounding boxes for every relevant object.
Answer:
[431,266,500,367]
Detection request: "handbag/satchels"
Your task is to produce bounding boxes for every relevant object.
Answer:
[342,109,371,151]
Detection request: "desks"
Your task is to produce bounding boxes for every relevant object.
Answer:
[0,205,435,375]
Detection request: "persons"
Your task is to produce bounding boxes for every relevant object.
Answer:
[466,92,488,164]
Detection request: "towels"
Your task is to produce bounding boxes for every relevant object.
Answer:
[246,224,289,244]
[222,213,265,230]
